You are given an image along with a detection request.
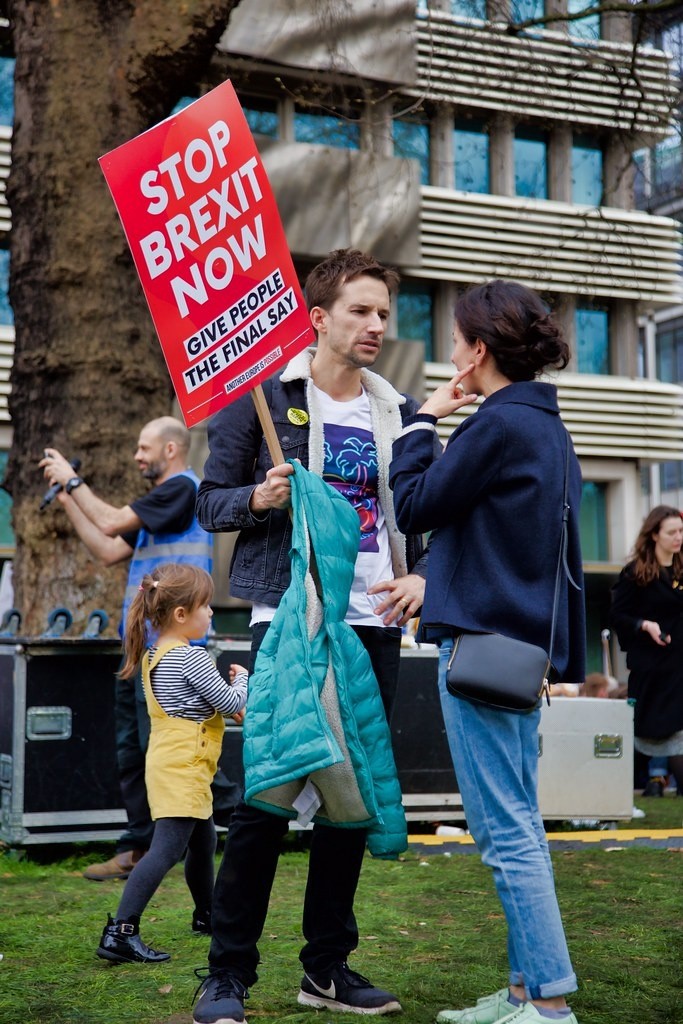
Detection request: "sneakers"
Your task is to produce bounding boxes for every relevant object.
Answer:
[296,959,402,1016]
[491,1003,577,1024]
[191,966,252,1024]
[435,986,525,1024]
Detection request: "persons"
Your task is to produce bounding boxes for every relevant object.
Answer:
[578,673,608,698]
[36,417,212,878]
[389,280,586,1024]
[94,563,247,963]
[192,247,434,1024]
[610,506,683,798]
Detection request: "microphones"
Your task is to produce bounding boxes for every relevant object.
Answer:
[39,459,82,510]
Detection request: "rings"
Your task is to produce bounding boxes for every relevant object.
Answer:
[401,599,408,605]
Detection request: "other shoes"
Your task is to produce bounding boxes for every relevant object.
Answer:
[85,852,137,881]
[640,776,667,798]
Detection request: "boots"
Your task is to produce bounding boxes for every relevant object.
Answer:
[192,910,211,936]
[97,912,171,964]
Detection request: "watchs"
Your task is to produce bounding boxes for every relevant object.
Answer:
[66,478,83,494]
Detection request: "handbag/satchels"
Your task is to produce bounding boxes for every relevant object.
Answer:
[447,631,550,715]
